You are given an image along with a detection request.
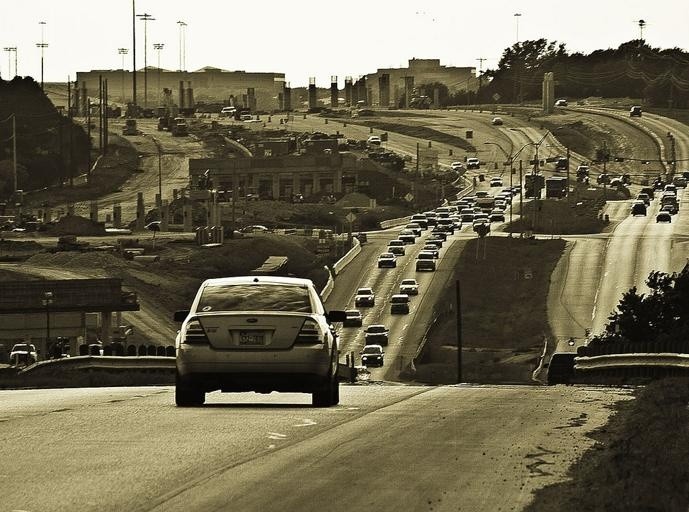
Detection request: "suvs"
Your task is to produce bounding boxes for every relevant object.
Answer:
[354,288,376,308]
[290,191,303,203]
[630,104,643,117]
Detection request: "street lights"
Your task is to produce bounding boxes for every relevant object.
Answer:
[42,291,54,359]
[3,7,196,144]
[636,19,647,104]
[482,139,541,233]
[514,13,522,74]
[508,127,565,230]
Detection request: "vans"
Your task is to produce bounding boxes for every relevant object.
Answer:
[389,294,411,315]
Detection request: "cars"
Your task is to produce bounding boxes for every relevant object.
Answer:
[338,97,374,116]
[524,151,688,223]
[367,136,382,146]
[221,106,253,121]
[466,158,479,170]
[8,343,41,364]
[387,240,406,256]
[491,118,505,126]
[364,325,390,347]
[377,252,397,268]
[555,100,569,106]
[489,176,503,187]
[399,278,419,296]
[398,184,530,272]
[343,309,363,327]
[169,273,345,409]
[358,345,385,367]
[451,161,463,171]
[232,225,272,237]
